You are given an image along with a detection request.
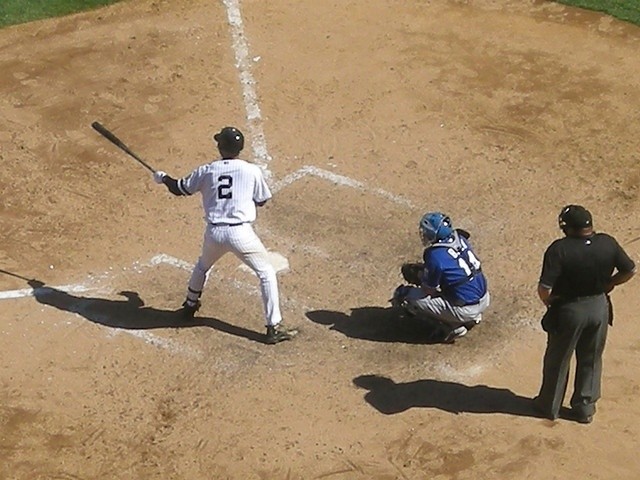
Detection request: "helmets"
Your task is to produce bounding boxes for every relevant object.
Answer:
[213,127,243,152]
[559,205,592,237]
[419,211,453,251]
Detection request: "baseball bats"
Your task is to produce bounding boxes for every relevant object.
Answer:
[91,121,156,175]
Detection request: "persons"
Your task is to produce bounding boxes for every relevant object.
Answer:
[152,126,298,345]
[532,204,636,424]
[390,212,491,343]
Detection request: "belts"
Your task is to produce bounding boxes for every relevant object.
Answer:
[211,222,241,227]
[460,300,480,305]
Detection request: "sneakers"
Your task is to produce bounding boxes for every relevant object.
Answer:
[531,396,559,420]
[440,326,468,343]
[577,413,593,423]
[265,324,299,344]
[182,303,201,321]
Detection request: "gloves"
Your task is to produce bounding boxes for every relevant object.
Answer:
[152,171,167,184]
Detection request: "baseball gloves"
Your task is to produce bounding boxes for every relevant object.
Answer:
[401,260,424,288]
